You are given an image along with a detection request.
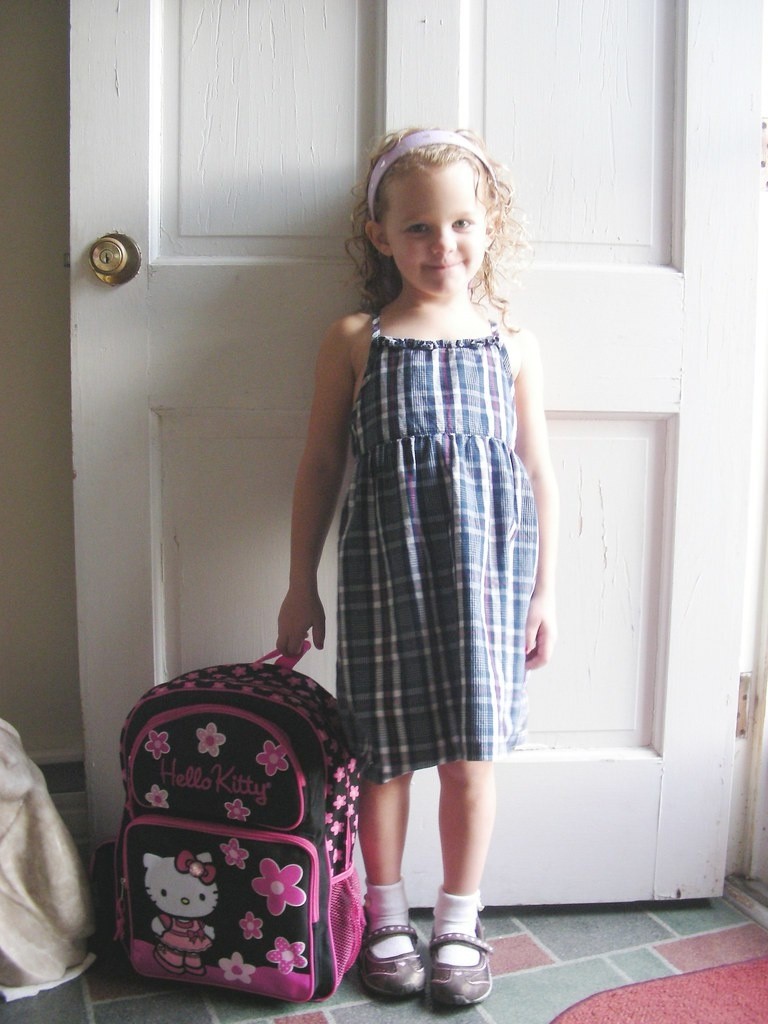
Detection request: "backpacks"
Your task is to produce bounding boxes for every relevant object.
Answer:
[88,641,365,1004]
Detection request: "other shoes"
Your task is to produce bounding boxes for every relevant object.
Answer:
[357,905,426,997]
[429,916,492,1006]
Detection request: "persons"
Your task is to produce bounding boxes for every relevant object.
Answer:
[276,128,556,1003]
